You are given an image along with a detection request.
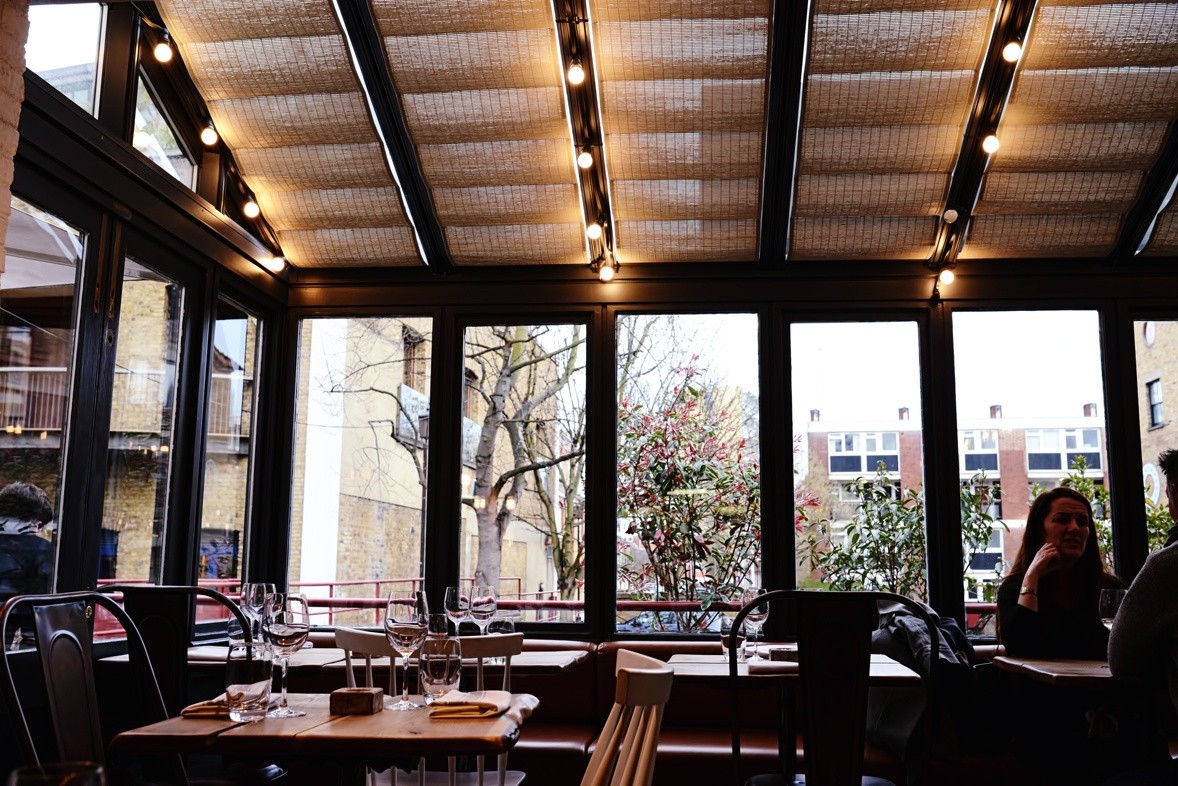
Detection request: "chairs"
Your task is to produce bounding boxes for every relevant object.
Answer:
[579,648,674,786]
[728,592,938,786]
[0,584,252,785]
[333,627,526,786]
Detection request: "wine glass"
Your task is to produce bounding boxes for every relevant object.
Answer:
[239,582,287,657]
[261,592,310,719]
[740,588,770,663]
[1098,589,1128,670]
[383,590,430,711]
[443,586,498,636]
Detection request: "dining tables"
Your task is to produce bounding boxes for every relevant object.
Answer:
[118,692,538,786]
[992,655,1156,760]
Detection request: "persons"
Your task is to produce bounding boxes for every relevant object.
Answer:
[1,481,57,646]
[1106,449,1178,742]
[995,488,1134,786]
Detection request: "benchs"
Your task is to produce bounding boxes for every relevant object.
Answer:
[99,632,1004,736]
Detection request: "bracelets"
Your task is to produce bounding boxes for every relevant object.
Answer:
[1019,586,1037,594]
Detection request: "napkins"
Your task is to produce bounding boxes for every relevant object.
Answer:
[428,689,511,719]
[180,679,271,717]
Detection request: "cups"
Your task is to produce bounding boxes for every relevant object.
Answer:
[226,642,274,724]
[720,617,746,662]
[486,616,515,664]
[227,606,250,649]
[418,613,462,706]
